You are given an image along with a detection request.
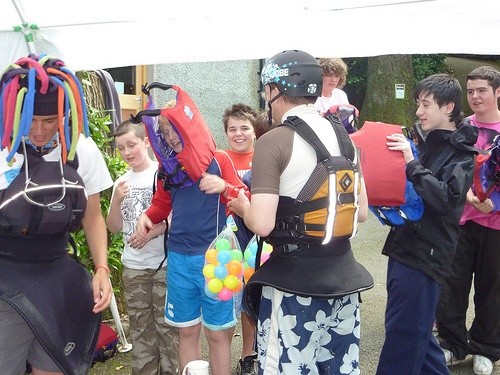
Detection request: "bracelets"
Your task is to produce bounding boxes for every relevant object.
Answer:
[94,265,110,274]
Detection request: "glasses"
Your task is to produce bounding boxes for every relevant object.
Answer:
[258,89,270,101]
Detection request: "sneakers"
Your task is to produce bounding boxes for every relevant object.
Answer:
[441,345,467,365]
[473,354,493,375]
[236,352,260,375]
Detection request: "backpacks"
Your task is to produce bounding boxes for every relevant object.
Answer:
[323,105,359,134]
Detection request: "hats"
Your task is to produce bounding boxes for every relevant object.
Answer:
[17,83,71,116]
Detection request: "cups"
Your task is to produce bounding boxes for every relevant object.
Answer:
[182,360,213,375]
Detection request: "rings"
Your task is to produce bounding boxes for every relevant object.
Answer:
[230,202,232,207]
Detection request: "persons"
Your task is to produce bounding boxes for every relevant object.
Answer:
[436,66,500,375]
[223,103,277,375]
[377,74,479,375]
[130,82,251,375]
[105,119,181,375]
[226,50,375,375]
[0,51,112,375]
[314,58,360,135]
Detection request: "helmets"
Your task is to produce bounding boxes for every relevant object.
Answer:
[262,50,323,97]
